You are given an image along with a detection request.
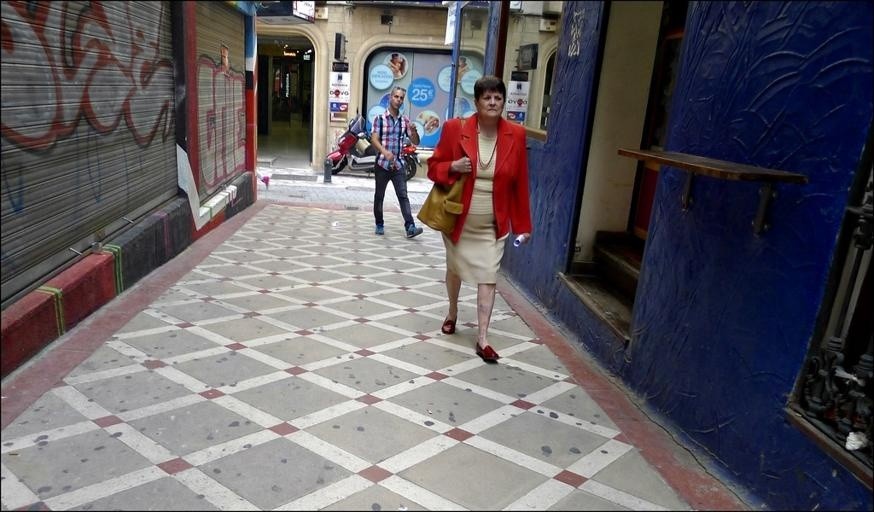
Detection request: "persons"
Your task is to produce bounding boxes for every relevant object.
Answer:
[427,76,532,361]
[371,86,424,237]
[388,51,405,79]
[458,56,469,80]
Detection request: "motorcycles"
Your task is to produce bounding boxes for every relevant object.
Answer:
[324,105,423,182]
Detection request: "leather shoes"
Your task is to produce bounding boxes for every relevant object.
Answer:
[476,342,499,360]
[442,316,457,334]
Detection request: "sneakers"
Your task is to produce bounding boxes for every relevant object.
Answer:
[407,224,423,238]
[375,224,384,234]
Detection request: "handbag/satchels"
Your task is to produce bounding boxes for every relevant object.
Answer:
[416,181,464,238]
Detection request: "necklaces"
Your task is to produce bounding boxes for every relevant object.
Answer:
[477,126,498,169]
